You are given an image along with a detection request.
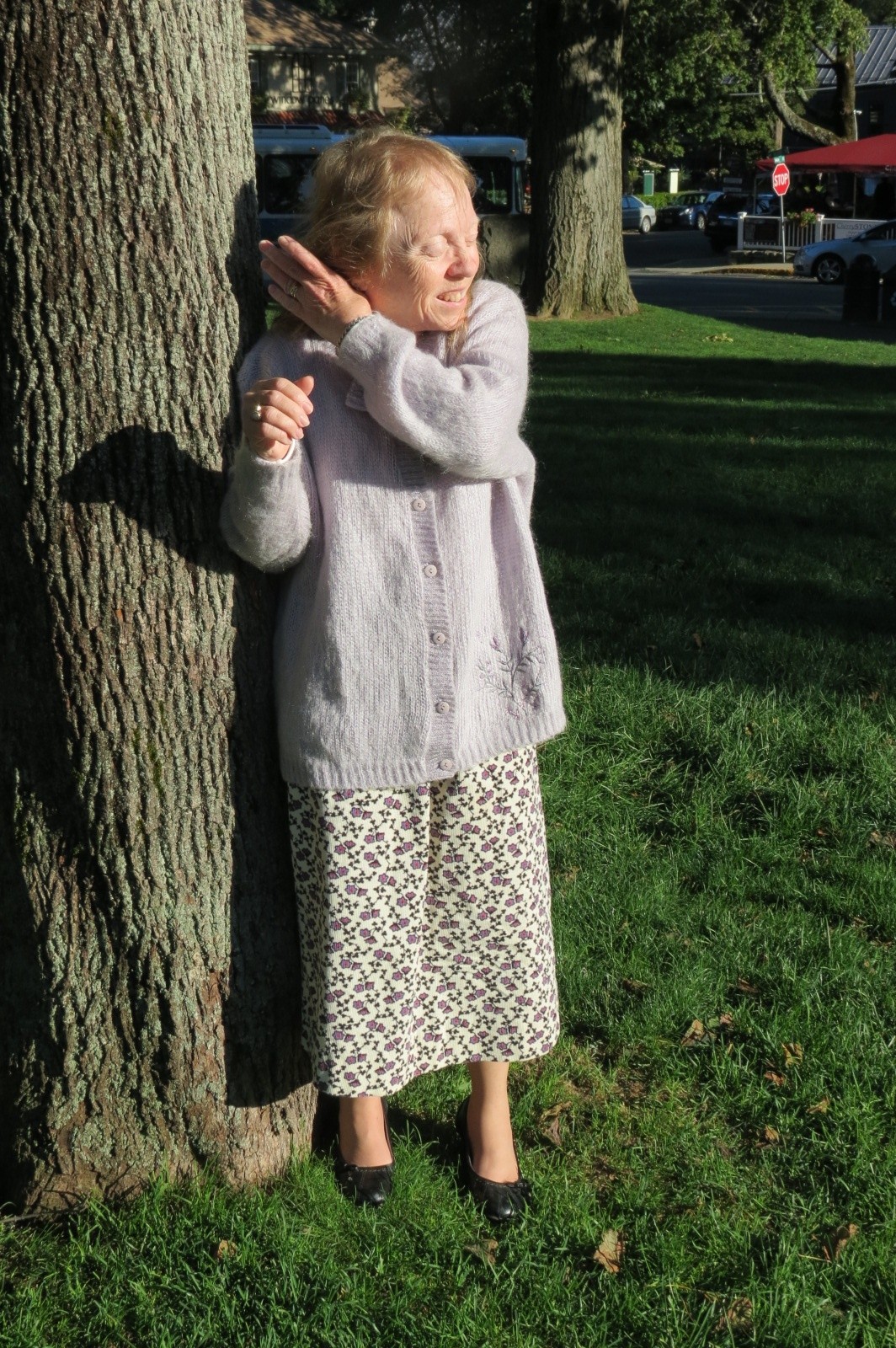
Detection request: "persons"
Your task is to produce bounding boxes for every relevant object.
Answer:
[221,123,568,1222]
[767,173,896,246]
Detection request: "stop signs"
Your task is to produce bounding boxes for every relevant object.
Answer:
[772,163,790,196]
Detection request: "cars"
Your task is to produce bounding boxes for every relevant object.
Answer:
[658,190,726,231]
[620,195,656,234]
[792,220,896,286]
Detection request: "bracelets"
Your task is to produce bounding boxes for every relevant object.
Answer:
[336,314,370,356]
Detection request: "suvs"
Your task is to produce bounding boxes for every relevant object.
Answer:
[704,192,808,253]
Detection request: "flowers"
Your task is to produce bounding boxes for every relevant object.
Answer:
[786,208,816,228]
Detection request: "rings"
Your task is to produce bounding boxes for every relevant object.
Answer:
[289,283,299,297]
[251,404,265,421]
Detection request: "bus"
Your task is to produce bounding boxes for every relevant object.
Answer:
[252,122,529,257]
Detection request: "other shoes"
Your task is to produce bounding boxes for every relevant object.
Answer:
[455,1095,531,1221]
[332,1098,396,1205]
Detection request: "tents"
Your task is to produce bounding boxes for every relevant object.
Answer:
[756,134,896,219]
